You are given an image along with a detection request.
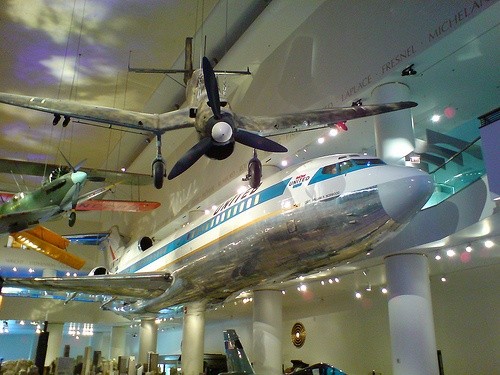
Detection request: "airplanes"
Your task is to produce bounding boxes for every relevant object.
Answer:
[0,148,128,234]
[0,152,435,324]
[0,36,419,192]
[1,158,162,212]
